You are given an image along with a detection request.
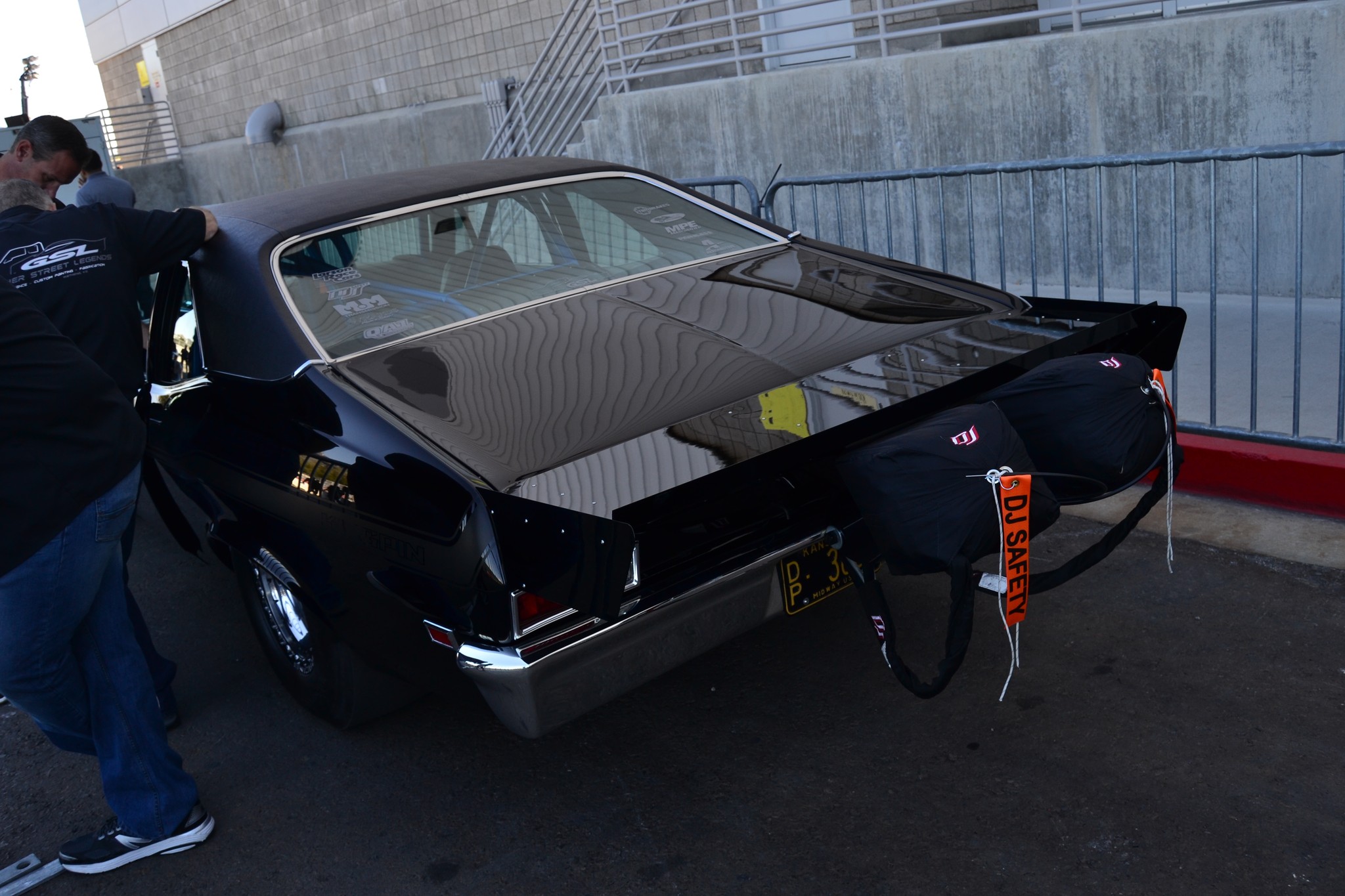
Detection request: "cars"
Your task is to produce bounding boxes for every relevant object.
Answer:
[130,153,1187,745]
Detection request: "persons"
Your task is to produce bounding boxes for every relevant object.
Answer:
[71,147,155,353]
[0,174,259,876]
[0,115,91,215]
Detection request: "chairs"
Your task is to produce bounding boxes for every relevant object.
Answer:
[284,240,608,352]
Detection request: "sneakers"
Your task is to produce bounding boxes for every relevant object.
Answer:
[56,796,215,875]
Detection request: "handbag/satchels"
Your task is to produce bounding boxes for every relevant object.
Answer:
[837,402,1065,703]
[982,347,1185,596]
